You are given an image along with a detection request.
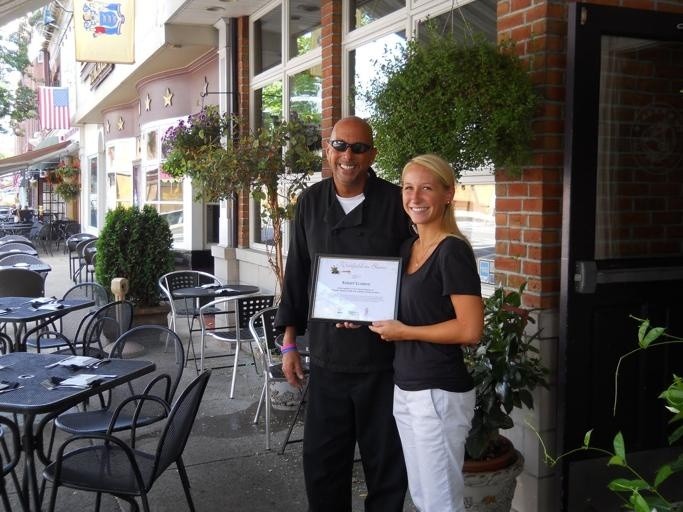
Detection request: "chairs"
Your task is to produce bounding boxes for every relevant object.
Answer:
[248,306,310,450]
[200,294,274,399]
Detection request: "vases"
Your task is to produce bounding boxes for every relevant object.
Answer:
[62,175,77,184]
[52,183,58,191]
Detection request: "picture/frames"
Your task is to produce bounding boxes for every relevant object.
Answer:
[307,253,403,327]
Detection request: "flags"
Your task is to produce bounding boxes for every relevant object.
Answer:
[37,86,71,130]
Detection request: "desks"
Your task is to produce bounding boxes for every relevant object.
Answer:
[174,284,260,377]
[275,334,316,455]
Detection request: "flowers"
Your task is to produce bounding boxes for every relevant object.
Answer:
[56,158,81,176]
[48,171,61,185]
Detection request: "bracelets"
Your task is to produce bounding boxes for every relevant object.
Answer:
[280,342,298,354]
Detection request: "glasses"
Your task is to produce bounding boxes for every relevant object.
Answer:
[326,138,374,154]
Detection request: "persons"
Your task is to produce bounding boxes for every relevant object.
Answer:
[335,154,486,512]
[270,115,413,512]
[14,192,21,209]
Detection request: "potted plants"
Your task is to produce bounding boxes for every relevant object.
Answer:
[161,111,328,411]
[462,281,549,512]
